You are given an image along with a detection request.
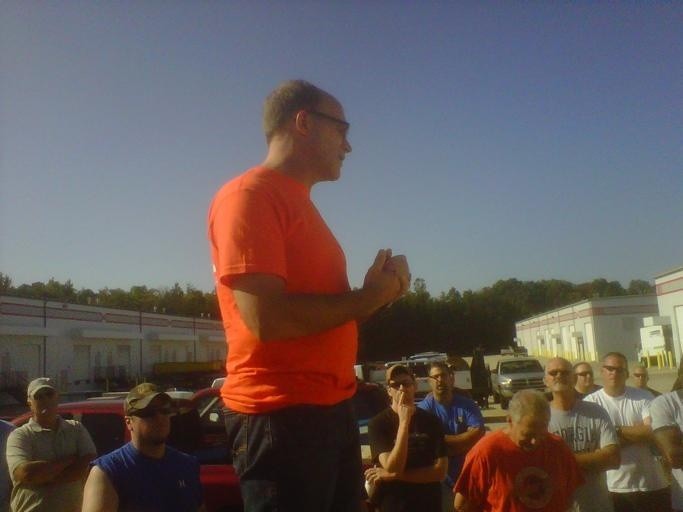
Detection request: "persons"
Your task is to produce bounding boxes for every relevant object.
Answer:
[451,388,588,512]
[649,357,683,512]
[542,357,621,511]
[631,365,662,396]
[207,79,410,511]
[581,351,673,512]
[363,364,450,512]
[417,361,486,512]
[574,362,605,400]
[79,383,207,511]
[5,377,99,512]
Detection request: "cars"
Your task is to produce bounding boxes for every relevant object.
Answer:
[354,345,554,410]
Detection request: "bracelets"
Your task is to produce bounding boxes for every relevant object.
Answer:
[616,425,621,437]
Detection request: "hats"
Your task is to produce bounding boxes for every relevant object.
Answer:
[27,377,59,403]
[123,383,172,415]
[386,364,415,382]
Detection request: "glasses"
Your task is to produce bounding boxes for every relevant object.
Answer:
[576,370,592,377]
[34,391,55,399]
[635,373,647,376]
[308,109,350,135]
[605,365,628,372]
[141,407,171,418]
[389,383,414,388]
[548,369,571,376]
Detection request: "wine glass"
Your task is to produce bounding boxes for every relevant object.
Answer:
[364,478,377,502]
